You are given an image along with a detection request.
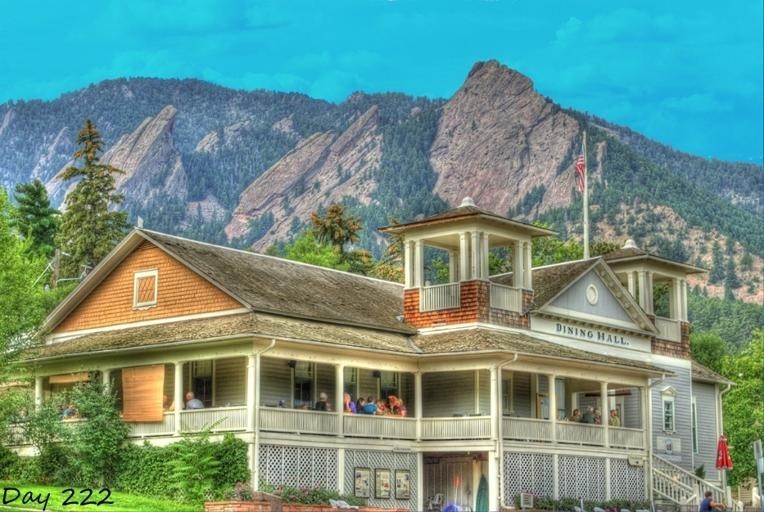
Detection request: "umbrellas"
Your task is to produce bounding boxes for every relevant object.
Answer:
[716,435,733,488]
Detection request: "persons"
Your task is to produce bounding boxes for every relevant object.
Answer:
[344,392,406,417]
[63,403,81,419]
[169,398,189,409]
[186,391,205,409]
[315,392,333,411]
[700,491,725,511]
[163,393,173,411]
[570,404,621,427]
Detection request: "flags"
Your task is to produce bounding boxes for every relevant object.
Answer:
[573,140,585,193]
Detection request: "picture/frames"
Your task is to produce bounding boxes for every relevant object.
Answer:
[354,467,411,499]
